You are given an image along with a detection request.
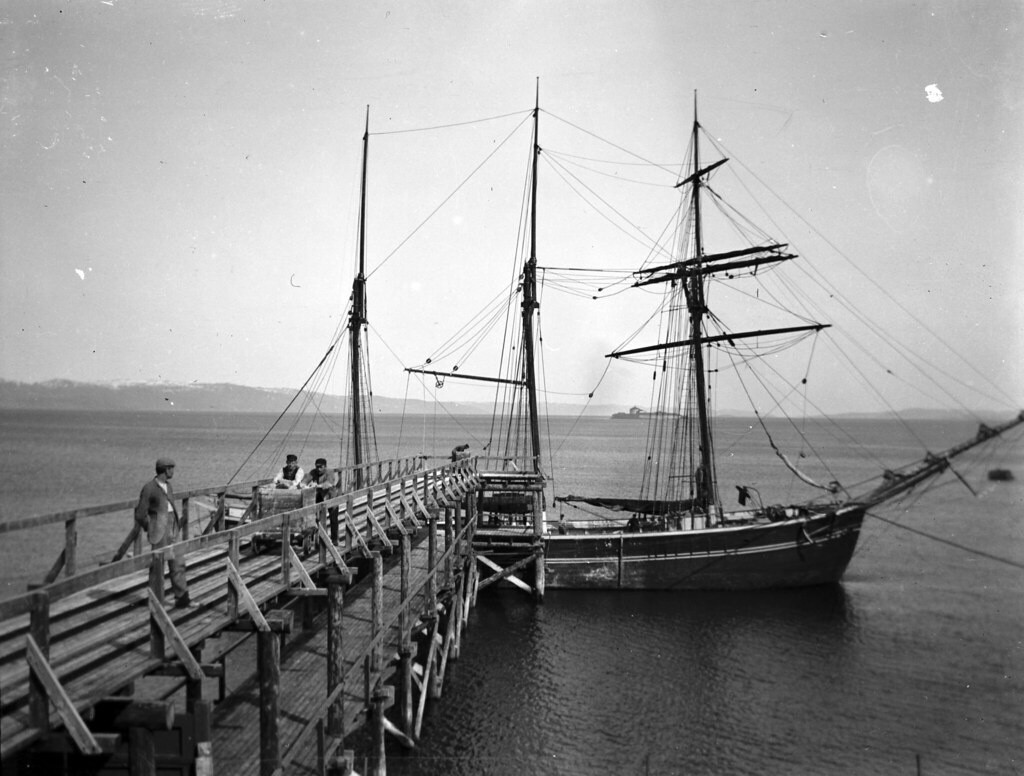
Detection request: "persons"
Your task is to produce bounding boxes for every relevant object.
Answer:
[298,458,339,546]
[273,455,303,488]
[134,457,200,608]
[452,444,470,462]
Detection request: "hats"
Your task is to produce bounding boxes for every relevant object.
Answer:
[156,457,176,468]
[286,455,297,461]
[315,458,326,466]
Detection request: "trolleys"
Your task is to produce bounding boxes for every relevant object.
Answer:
[250,480,318,557]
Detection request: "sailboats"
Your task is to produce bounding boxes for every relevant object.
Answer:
[396,75,1023,591]
[192,103,384,535]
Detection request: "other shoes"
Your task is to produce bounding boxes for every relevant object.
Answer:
[331,543,338,547]
[185,601,200,608]
